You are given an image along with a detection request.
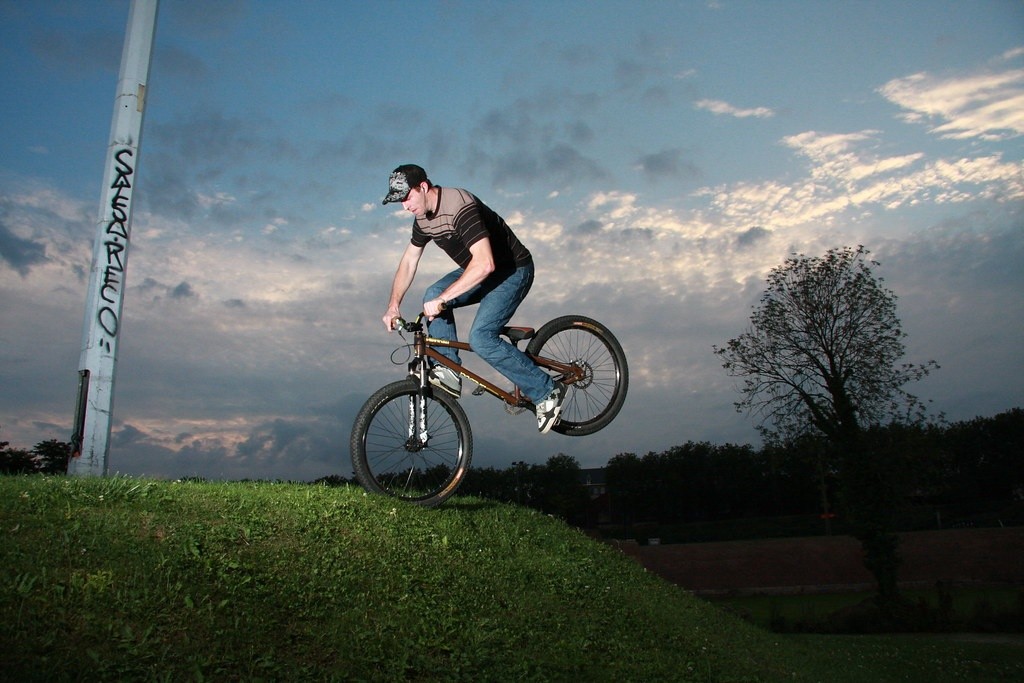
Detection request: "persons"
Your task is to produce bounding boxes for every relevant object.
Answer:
[382,163,570,435]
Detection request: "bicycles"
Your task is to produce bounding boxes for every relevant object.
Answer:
[349,302,628,511]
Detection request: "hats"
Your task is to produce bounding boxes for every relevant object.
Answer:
[382,164,427,205]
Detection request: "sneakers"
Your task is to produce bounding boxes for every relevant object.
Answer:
[536,381,569,434]
[415,365,463,398]
